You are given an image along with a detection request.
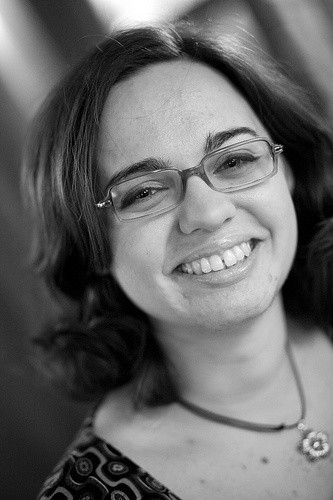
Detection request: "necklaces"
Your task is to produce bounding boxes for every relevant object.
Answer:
[172,340,332,462]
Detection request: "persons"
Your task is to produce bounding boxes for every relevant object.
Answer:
[24,24,333,500]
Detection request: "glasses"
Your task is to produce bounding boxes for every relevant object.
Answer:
[97,137,287,225]
